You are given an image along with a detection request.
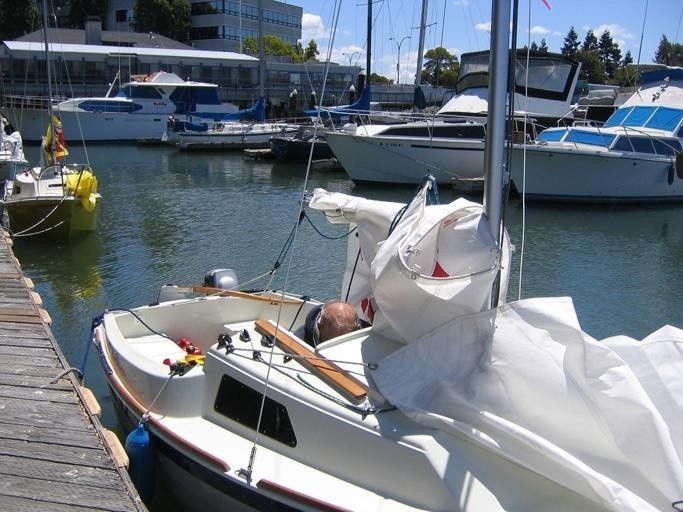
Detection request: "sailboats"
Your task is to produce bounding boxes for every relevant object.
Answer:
[90,0,683,512]
[0,0,97,238]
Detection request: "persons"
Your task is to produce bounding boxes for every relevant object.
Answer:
[293,297,371,349]
